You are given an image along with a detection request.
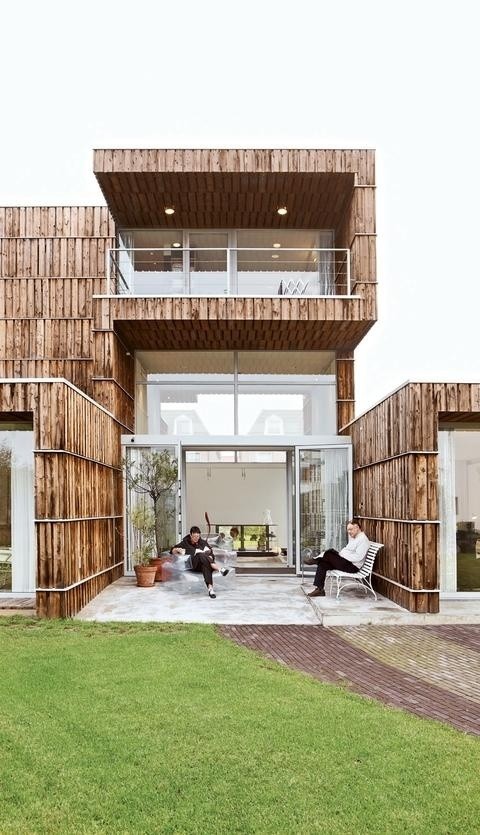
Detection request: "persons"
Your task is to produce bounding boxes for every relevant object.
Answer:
[169,526,230,599]
[303,521,371,596]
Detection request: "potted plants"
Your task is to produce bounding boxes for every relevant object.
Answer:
[119,448,179,589]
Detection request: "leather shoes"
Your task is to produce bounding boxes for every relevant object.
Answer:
[308,588,325,596]
[303,557,322,565]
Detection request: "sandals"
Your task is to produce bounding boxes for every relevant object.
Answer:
[221,569,229,576]
[209,589,216,598]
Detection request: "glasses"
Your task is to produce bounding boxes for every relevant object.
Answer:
[192,535,200,537]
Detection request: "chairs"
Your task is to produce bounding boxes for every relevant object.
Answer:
[162,532,238,598]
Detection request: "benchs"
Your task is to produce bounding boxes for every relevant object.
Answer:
[323,541,385,604]
